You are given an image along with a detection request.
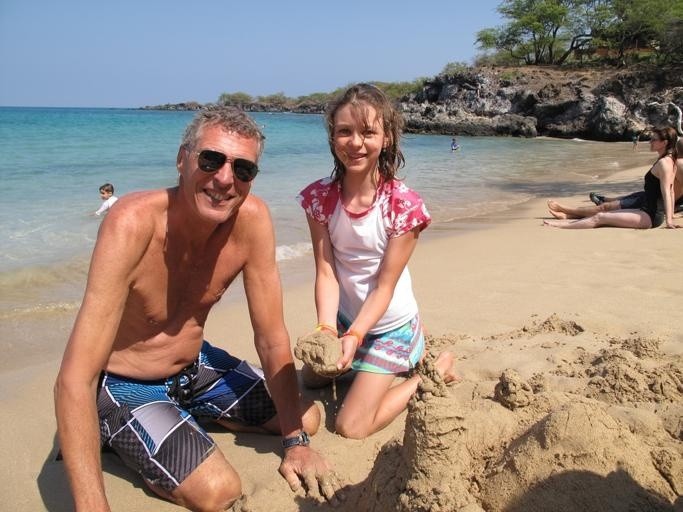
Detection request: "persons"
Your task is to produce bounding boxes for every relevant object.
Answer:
[296,81,461,438]
[91,183,120,213]
[633,130,642,146]
[451,135,460,151]
[542,124,681,229]
[54,104,348,511]
[588,123,683,215]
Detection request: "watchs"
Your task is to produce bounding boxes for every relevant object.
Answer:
[280,433,309,449]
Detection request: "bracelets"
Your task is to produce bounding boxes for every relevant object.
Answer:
[316,324,338,335]
[341,330,364,345]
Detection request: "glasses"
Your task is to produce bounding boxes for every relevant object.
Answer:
[649,136,657,143]
[197,150,258,182]
